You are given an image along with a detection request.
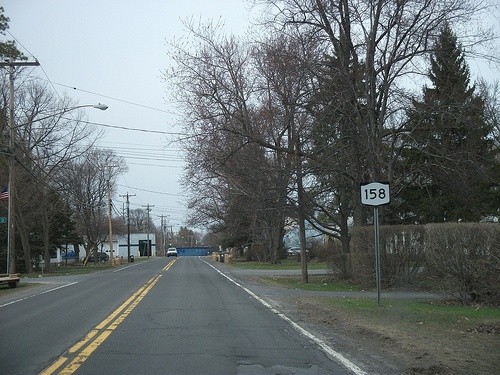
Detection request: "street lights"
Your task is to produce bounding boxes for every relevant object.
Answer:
[7,102,109,277]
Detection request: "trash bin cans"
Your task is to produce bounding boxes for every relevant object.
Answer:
[219,253,225,262]
[130,255,134,262]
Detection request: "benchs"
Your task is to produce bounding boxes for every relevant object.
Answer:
[0,277,20,288]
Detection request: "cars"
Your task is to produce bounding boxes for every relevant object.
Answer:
[286,246,310,256]
[60,249,76,259]
[165,247,178,257]
[80,251,109,264]
